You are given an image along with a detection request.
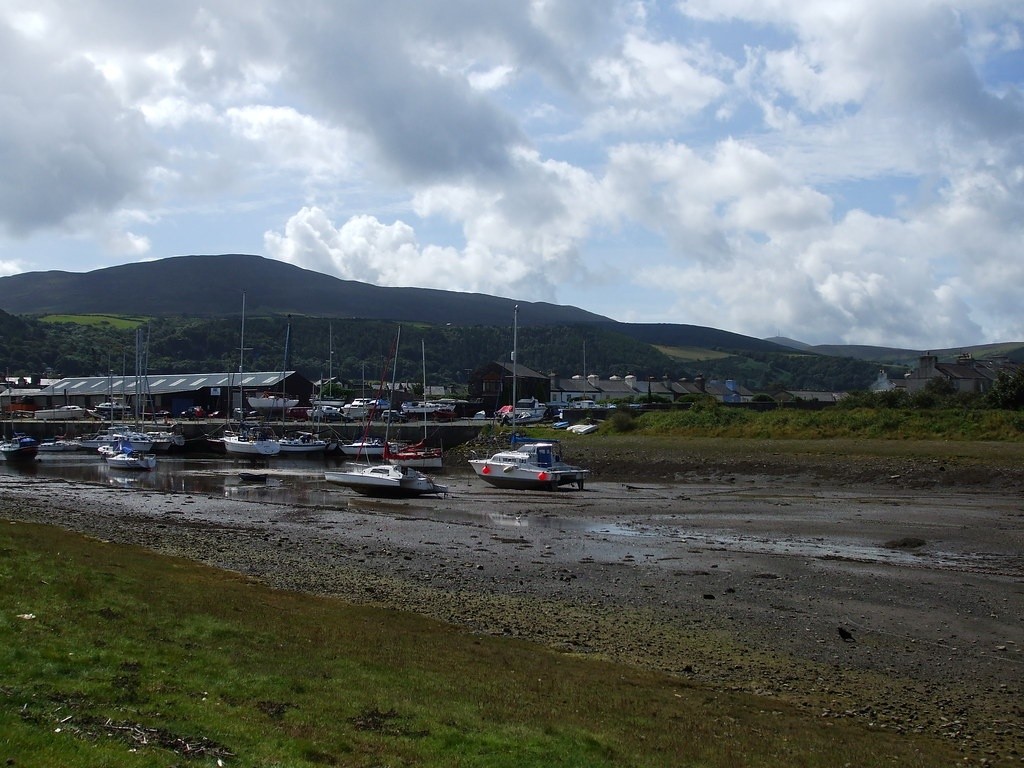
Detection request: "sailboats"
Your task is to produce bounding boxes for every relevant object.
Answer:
[0,324,226,475]
[222,292,609,497]
